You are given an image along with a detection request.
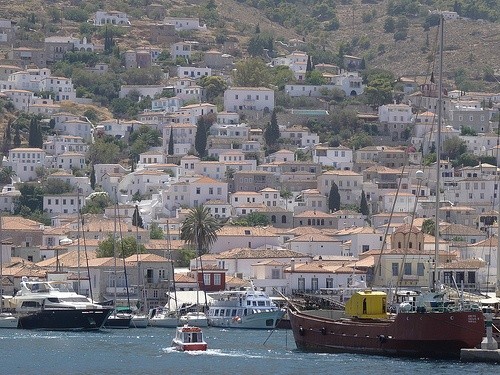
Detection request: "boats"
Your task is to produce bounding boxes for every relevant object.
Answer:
[0,186,500,332]
[275,14,500,357]
[172,324,207,352]
